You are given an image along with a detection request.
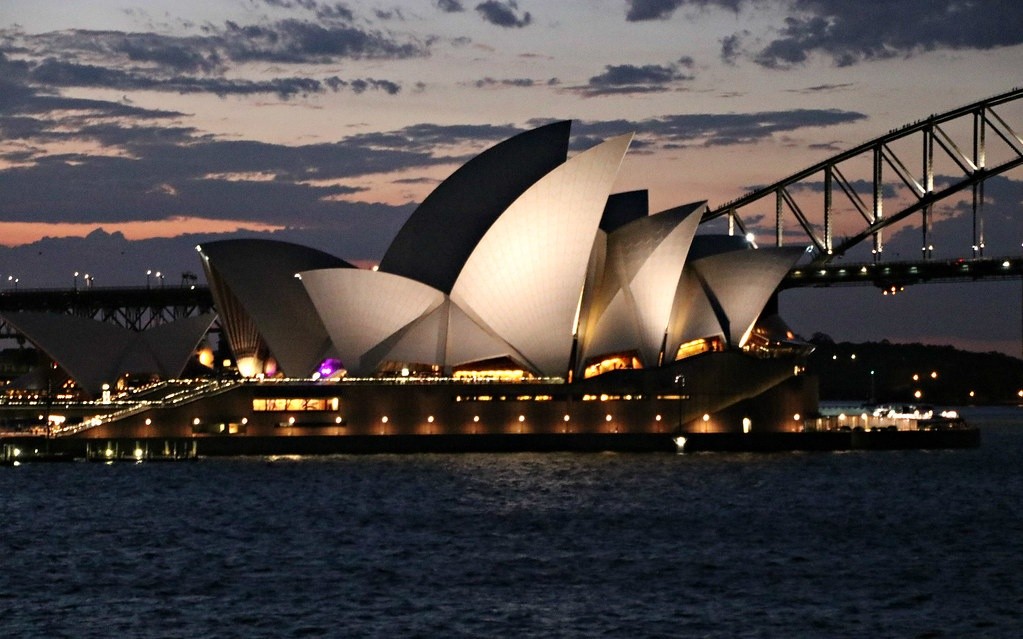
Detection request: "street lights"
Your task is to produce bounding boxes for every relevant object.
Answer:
[95,419,101,437]
[518,415,525,434]
[980,243,984,256]
[427,416,433,434]
[145,418,151,438]
[8,275,12,293]
[14,278,18,291]
[381,416,388,434]
[655,414,660,433]
[872,249,876,261]
[794,413,800,432]
[289,417,294,436]
[473,416,479,433]
[878,247,882,261]
[928,245,933,259]
[155,271,161,285]
[73,271,78,289]
[84,273,88,286]
[703,413,709,432]
[745,231,754,249]
[921,246,926,260]
[563,414,569,433]
[193,418,200,434]
[838,413,847,427]
[242,417,247,434]
[335,415,341,435]
[100,384,110,403]
[827,249,833,263]
[972,245,978,257]
[145,270,151,289]
[861,412,867,430]
[606,414,612,433]
